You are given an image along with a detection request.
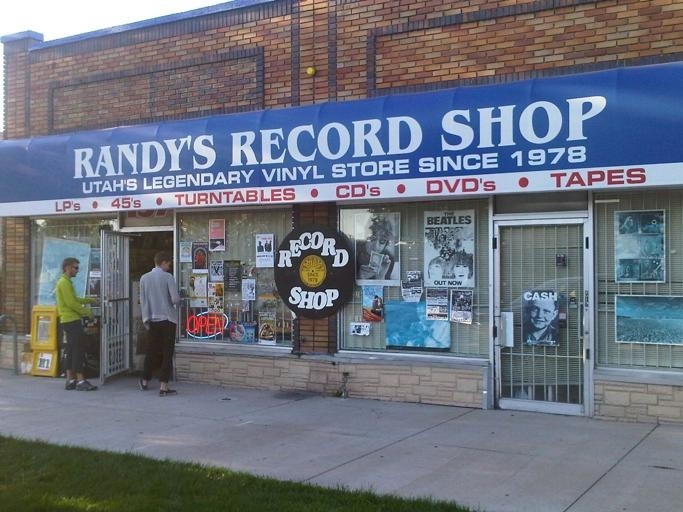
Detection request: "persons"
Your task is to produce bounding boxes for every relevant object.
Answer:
[54,257,100,391]
[369,294,380,317]
[134,249,181,396]
[427,221,473,281]
[446,252,474,283]
[617,216,665,281]
[264,240,268,251]
[523,289,558,346]
[354,212,394,282]
[267,240,271,251]
[257,241,264,252]
[427,255,445,281]
[374,297,382,312]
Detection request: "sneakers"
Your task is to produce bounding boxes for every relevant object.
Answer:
[138,377,147,390]
[65,379,98,391]
[159,390,177,396]
